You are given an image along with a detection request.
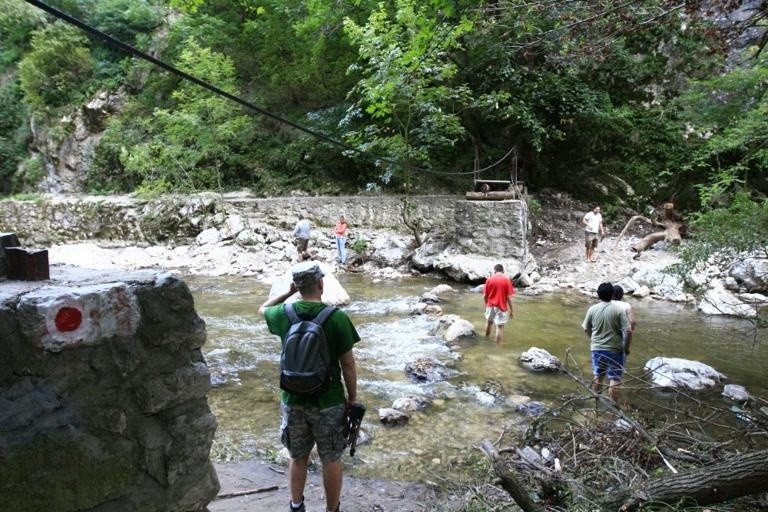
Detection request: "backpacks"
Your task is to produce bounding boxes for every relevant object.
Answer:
[278,303,340,397]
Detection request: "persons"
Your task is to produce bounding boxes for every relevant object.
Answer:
[293,214,312,263]
[258,261,362,512]
[611,283,636,359]
[581,205,605,262]
[581,282,633,409]
[482,263,514,344]
[334,215,347,265]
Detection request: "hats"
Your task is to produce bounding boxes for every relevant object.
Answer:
[291,261,326,291]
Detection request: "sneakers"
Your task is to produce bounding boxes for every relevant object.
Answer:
[289,495,306,512]
